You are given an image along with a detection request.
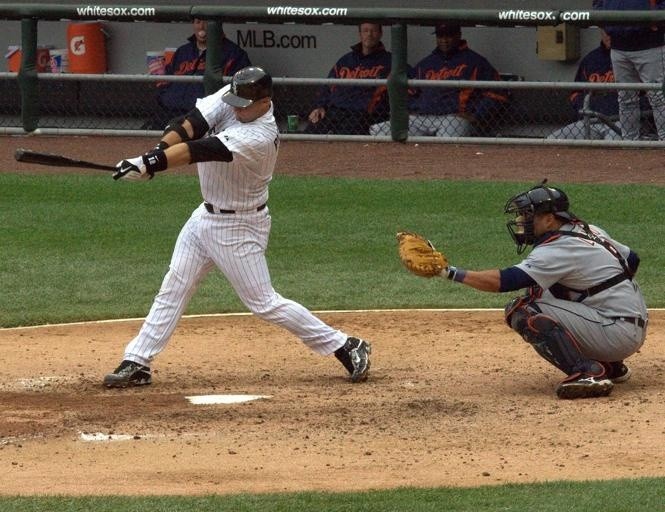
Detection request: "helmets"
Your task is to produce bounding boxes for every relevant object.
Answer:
[504,184,570,244]
[220,64,274,109]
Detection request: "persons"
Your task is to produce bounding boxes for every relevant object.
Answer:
[148,16,251,130]
[367,21,510,137]
[99,66,374,387]
[545,28,650,142]
[305,24,413,135]
[394,182,649,402]
[591,0,665,143]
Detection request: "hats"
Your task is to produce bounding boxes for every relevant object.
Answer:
[431,21,463,36]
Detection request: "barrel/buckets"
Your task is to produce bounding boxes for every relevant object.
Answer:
[36,43,57,73]
[66,21,112,74]
[164,47,178,67]
[143,50,165,74]
[5,45,23,73]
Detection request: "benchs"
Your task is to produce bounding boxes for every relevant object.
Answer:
[0,69,646,127]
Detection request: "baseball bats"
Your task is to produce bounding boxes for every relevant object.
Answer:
[15,147,121,173]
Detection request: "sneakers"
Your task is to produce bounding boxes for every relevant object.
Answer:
[556,376,615,400]
[604,359,633,384]
[344,335,370,382]
[105,359,152,389]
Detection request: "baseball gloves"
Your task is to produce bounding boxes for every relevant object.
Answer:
[397,232,448,279]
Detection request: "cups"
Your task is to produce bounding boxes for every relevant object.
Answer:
[287,115,298,132]
[146,51,166,76]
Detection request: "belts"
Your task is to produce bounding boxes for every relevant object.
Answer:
[610,312,649,328]
[202,201,267,214]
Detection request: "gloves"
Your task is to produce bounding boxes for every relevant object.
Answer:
[111,151,160,184]
